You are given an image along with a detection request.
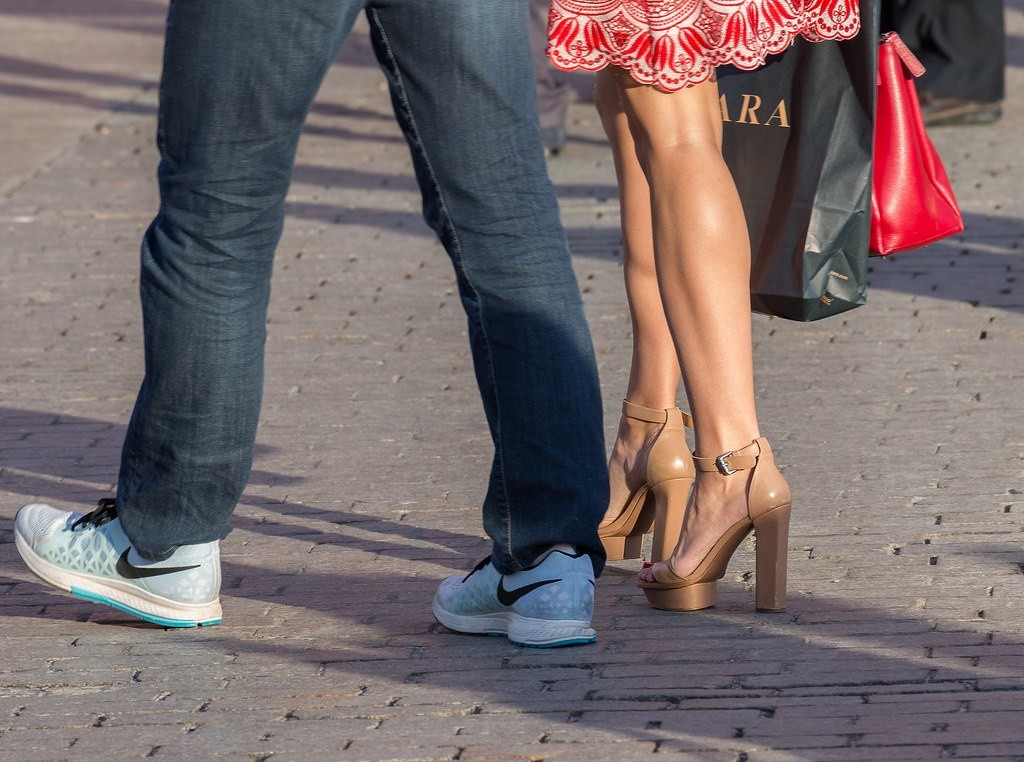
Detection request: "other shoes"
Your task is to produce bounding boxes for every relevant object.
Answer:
[542,125,566,151]
[915,86,1003,126]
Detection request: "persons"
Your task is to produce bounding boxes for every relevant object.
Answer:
[13,0,610,648]
[882,2,1005,127]
[544,0,861,611]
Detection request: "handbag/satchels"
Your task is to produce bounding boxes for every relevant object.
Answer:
[715,0,881,321]
[868,31,964,259]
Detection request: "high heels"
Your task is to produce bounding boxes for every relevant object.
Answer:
[635,437,792,612]
[597,399,694,563]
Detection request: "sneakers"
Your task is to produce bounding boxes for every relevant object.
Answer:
[14,497,223,628]
[432,550,597,648]
